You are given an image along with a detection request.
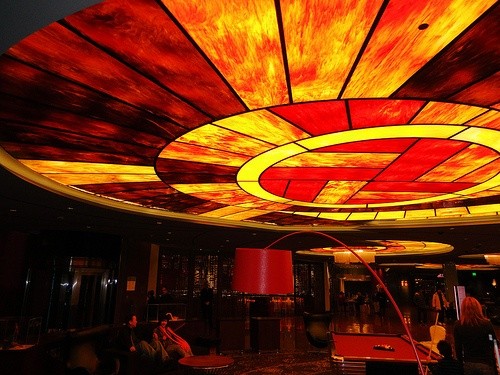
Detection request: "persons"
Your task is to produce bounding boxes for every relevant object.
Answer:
[433,339,464,375]
[364,301,377,320]
[453,297,500,375]
[355,293,364,322]
[200,282,214,331]
[376,289,387,320]
[432,286,449,326]
[153,313,193,358]
[114,312,154,355]
[414,288,429,324]
[159,286,174,304]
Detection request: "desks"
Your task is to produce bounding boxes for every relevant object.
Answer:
[216,318,245,355]
[250,317,281,353]
[327,331,443,375]
[184,320,209,355]
[178,355,234,375]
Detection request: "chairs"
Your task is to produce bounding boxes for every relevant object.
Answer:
[420,325,446,354]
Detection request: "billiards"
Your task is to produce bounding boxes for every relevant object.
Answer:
[374,344,392,350]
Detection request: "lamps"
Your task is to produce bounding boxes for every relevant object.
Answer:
[232,231,426,375]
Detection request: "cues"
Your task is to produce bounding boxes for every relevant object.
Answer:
[424,312,439,375]
